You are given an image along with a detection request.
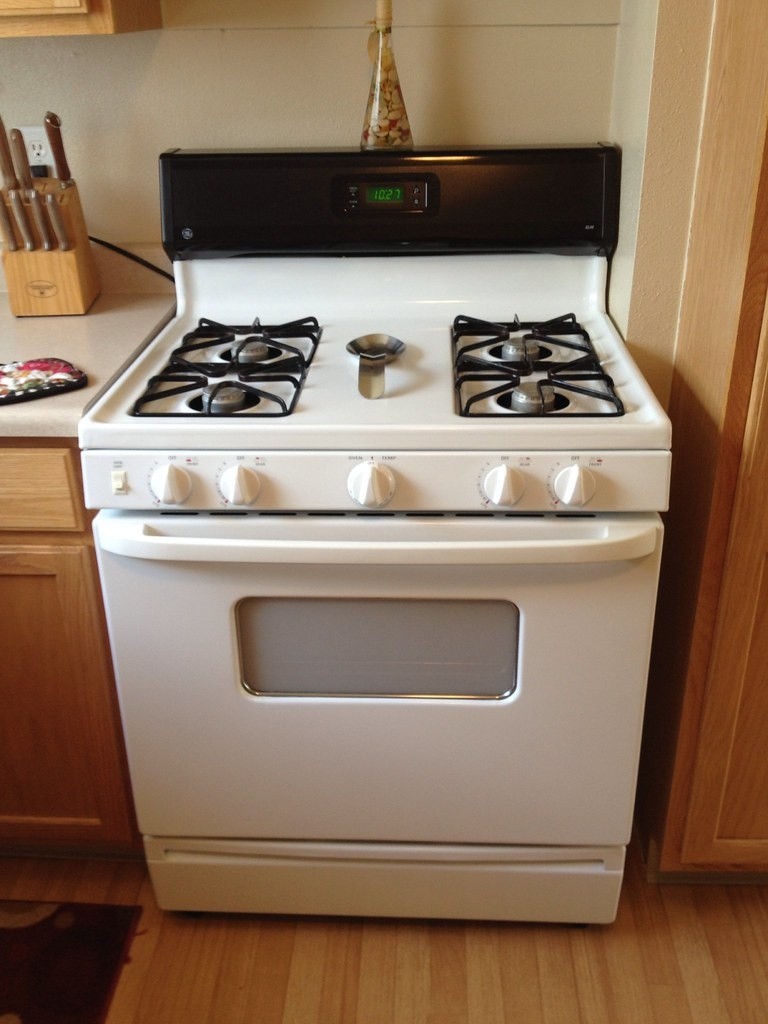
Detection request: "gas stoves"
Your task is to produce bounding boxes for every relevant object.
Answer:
[73,144,671,512]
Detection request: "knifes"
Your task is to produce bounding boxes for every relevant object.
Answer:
[0,111,71,251]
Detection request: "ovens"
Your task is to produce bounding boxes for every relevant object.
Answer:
[90,513,664,925]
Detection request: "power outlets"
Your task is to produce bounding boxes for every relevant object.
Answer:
[15,125,58,179]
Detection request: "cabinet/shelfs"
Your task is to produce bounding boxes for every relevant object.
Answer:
[0,0,164,38]
[638,132,765,888]
[0,437,146,860]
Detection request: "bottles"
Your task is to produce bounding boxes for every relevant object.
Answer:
[360,1,415,150]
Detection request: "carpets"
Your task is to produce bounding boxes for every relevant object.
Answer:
[1,896,144,1022]
